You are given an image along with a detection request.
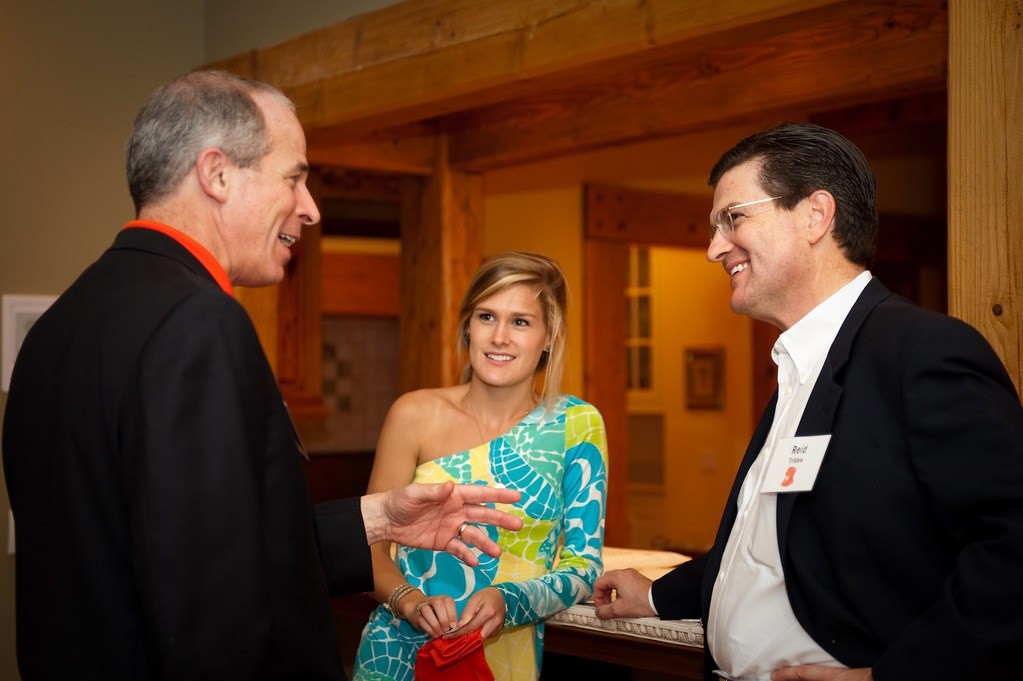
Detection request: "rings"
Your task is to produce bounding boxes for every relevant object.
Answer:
[416,602,427,616]
[458,523,470,535]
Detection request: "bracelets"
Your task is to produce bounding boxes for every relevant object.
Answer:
[387,583,421,619]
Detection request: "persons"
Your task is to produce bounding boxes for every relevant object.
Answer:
[594,122,1022,680]
[353,252,610,681]
[1,69,525,681]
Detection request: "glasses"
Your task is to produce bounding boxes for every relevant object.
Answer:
[709,196,781,242]
